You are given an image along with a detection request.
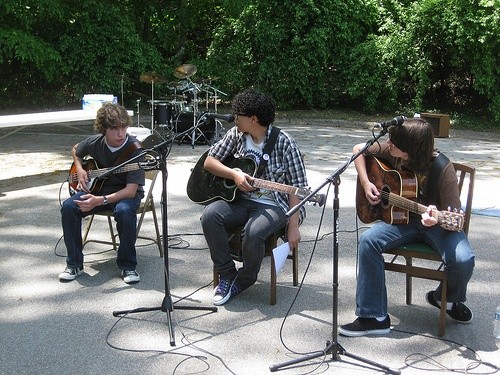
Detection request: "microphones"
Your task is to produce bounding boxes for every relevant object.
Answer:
[208,113,235,123]
[376,116,405,129]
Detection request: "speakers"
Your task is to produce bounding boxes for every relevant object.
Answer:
[421,113,450,138]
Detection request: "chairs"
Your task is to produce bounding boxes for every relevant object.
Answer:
[381,162,476,336]
[81,154,163,260]
[206,215,299,305]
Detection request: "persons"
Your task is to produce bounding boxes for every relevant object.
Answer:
[58,103,145,283]
[200,89,308,305]
[338,117,473,336]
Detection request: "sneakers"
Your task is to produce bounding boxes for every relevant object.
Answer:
[229,283,241,298]
[337,314,390,336]
[212,271,239,305]
[426,291,473,323]
[59,265,84,280]
[121,269,141,283]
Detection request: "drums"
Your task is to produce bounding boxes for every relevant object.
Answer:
[170,101,187,115]
[150,102,173,127]
[173,111,215,143]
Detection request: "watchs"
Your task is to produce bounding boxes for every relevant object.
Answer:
[103,195,108,205]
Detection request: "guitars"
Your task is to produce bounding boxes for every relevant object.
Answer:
[68,157,161,196]
[186,147,326,207]
[355,156,465,232]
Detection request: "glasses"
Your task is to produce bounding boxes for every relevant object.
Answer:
[233,112,250,118]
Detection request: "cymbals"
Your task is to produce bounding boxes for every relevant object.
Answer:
[132,90,150,101]
[173,64,197,79]
[193,76,222,83]
[140,73,165,84]
[113,73,135,81]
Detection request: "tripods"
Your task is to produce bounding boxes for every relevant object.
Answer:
[97,74,226,345]
[269,129,403,375]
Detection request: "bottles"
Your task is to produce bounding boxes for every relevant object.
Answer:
[493,305,500,339]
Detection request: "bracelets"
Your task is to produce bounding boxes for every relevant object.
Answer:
[437,222,442,225]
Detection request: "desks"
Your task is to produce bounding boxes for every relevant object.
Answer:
[421,113,449,138]
[0,108,134,140]
